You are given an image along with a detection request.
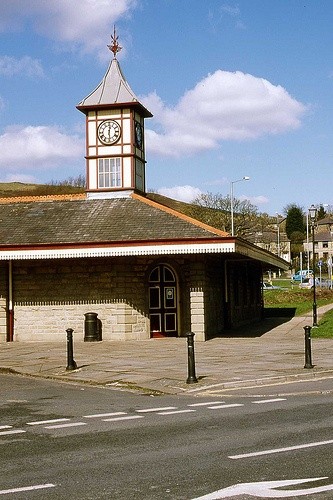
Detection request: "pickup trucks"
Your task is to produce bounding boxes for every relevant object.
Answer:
[298,277,333,290]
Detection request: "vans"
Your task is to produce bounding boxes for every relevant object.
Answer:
[293,270,314,281]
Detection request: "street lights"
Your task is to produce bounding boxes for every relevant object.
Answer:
[308,204,318,331]
[230,176,251,237]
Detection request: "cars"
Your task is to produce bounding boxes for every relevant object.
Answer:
[260,281,282,290]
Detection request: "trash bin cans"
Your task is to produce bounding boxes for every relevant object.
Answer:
[82,312,101,342]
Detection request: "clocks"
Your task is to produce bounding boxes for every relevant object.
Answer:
[135,122,142,147]
[97,119,121,145]
